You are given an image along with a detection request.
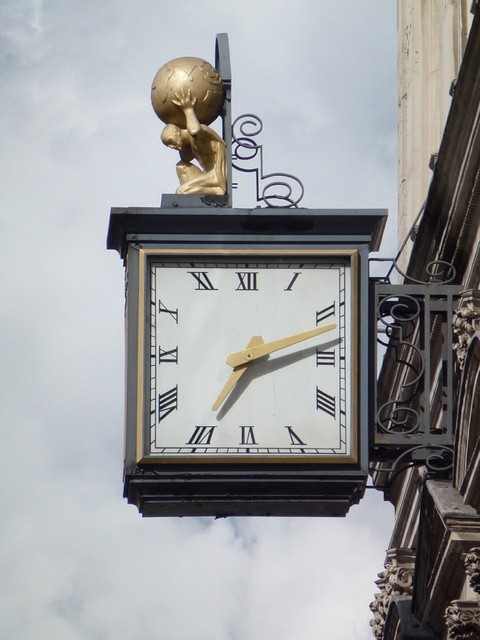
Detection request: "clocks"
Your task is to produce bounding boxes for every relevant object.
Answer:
[136,248,359,466]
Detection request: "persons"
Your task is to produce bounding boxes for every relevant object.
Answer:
[161,88,226,195]
